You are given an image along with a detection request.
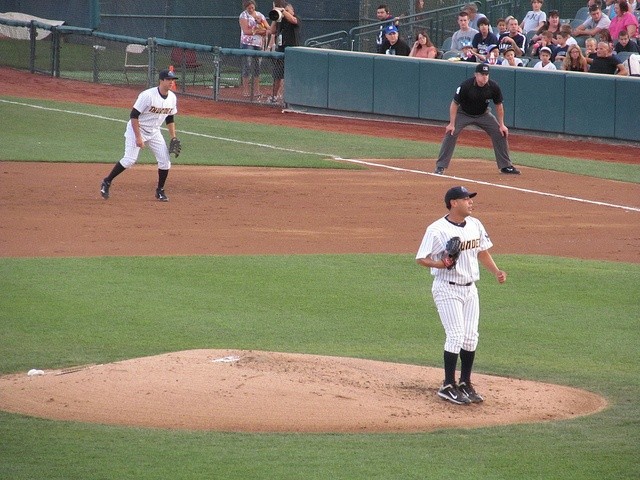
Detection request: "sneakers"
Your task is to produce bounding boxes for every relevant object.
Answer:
[155,187,168,202]
[434,166,445,175]
[100,178,112,200]
[458,378,484,403]
[436,380,471,405]
[501,165,520,175]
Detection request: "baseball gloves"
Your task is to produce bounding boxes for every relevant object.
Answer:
[440,237,463,272]
[169,137,183,159]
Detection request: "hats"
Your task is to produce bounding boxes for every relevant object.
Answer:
[444,186,477,204]
[159,70,179,79]
[486,45,498,54]
[460,40,473,49]
[475,64,490,74]
[540,47,552,55]
[503,46,515,56]
[386,25,398,35]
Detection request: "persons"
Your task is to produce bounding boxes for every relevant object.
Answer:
[584,38,598,61]
[376,4,400,54]
[504,15,522,33]
[607,0,616,20]
[451,13,479,55]
[472,18,498,63]
[396,0,424,34]
[585,41,628,76]
[415,186,510,406]
[466,44,487,68]
[561,43,588,72]
[604,0,613,9]
[434,64,523,177]
[378,24,411,56]
[100,70,182,201]
[553,31,570,62]
[614,31,639,52]
[537,30,561,61]
[559,25,578,46]
[409,31,436,59]
[496,18,508,35]
[271,0,302,102]
[587,0,602,12]
[460,2,493,34]
[571,5,611,44]
[609,0,639,41]
[239,0,271,98]
[483,44,510,66]
[519,0,546,34]
[627,0,638,13]
[500,48,524,67]
[252,16,265,35]
[499,19,527,58]
[533,47,557,70]
[458,40,476,63]
[529,9,561,48]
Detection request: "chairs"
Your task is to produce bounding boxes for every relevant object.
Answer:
[431,6,640,74]
[171,46,207,88]
[121,43,156,91]
